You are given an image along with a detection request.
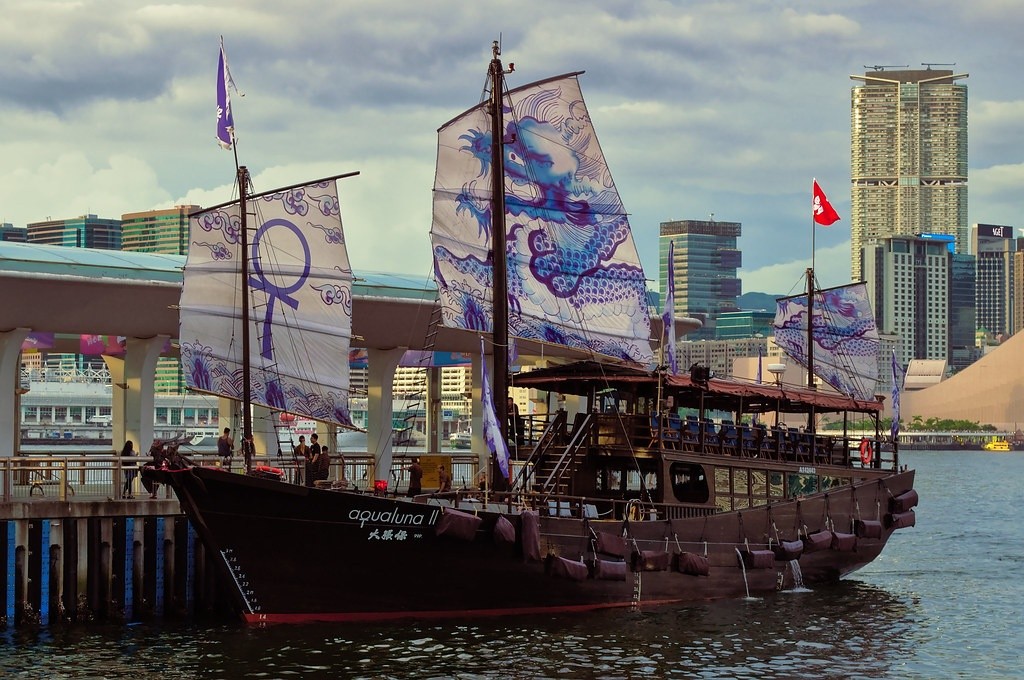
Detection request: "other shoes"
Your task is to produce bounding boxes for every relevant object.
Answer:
[127,495,135,499]
[122,495,126,499]
[149,496,157,499]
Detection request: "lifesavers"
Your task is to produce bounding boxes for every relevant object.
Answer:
[860,441,872,464]
[256,465,284,477]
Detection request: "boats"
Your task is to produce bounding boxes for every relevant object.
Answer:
[278,411,369,450]
[980,436,1011,452]
[188,433,220,447]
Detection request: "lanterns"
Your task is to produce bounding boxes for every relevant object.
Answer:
[280,412,295,422]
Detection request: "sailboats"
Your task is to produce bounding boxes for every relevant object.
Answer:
[137,23,920,626]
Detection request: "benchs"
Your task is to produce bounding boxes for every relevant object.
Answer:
[27,465,75,496]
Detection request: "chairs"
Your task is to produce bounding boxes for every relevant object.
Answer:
[649,411,833,465]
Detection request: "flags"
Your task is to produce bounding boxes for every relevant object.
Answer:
[813,180,841,227]
[892,354,900,442]
[480,350,510,479]
[756,350,762,420]
[664,243,678,375]
[216,45,234,151]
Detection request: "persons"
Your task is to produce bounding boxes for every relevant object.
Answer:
[554,393,566,411]
[144,439,167,499]
[401,457,423,496]
[434,464,450,493]
[308,446,330,487]
[507,397,525,445]
[751,419,756,427]
[294,435,309,484]
[121,441,139,499]
[309,434,319,474]
[480,471,486,490]
[217,427,234,471]
